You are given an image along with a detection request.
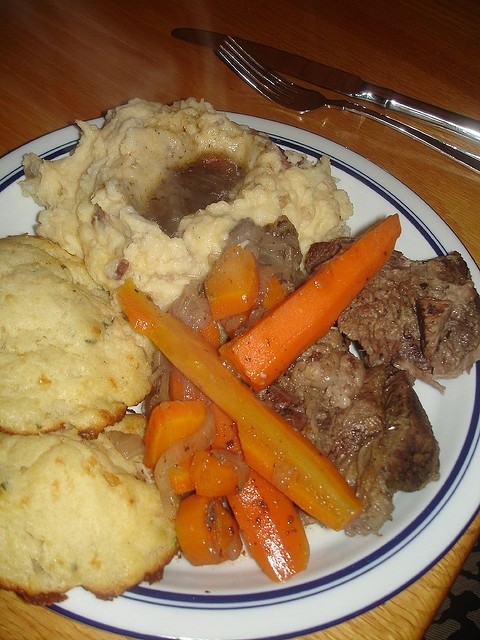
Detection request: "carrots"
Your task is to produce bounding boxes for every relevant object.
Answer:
[117,212,402,582]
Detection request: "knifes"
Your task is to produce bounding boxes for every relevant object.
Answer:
[171,28,479,141]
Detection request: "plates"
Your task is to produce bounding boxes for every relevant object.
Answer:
[0,109,479,639]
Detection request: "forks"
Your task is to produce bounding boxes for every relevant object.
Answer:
[211,33,479,174]
